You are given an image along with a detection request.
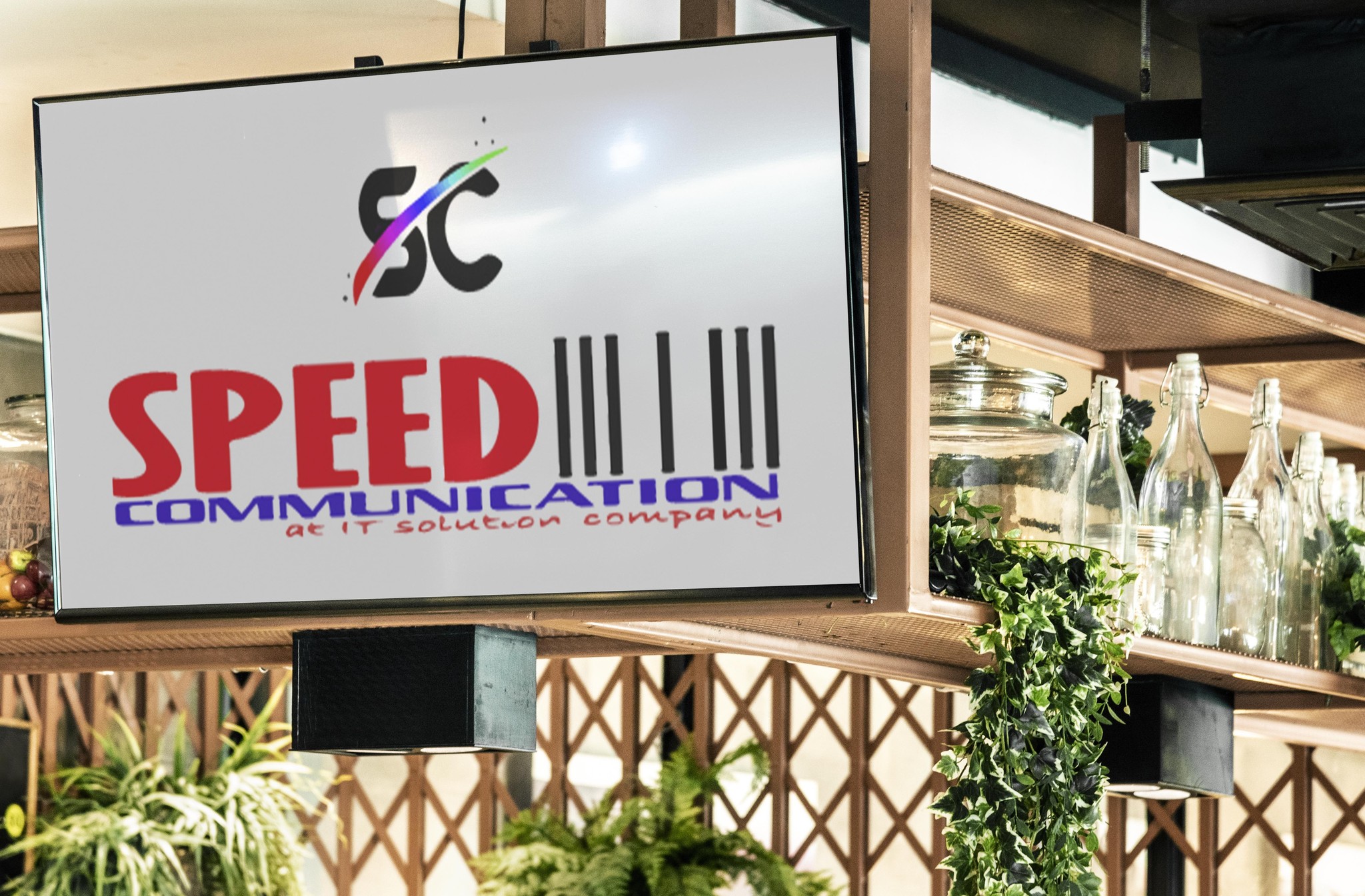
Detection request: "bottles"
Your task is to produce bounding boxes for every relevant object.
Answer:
[1289,429,1365,678]
[1086,522,1169,634]
[1218,498,1267,655]
[1075,374,1138,626]
[1139,352,1223,646]
[1230,377,1304,665]
